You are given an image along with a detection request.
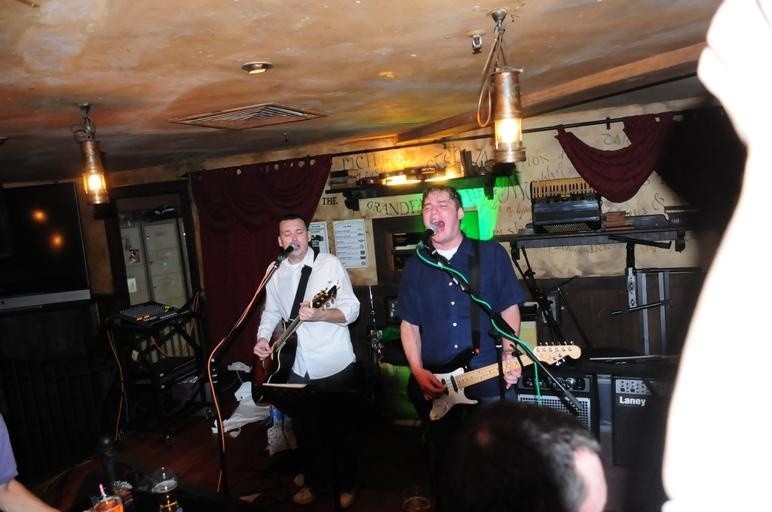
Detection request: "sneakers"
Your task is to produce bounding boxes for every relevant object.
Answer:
[332,491,355,509]
[293,487,316,504]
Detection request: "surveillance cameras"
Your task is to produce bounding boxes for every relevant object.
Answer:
[469,35,483,53]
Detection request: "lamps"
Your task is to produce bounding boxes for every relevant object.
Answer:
[488,64,526,163]
[79,141,109,205]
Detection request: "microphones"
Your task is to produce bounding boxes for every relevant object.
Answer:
[276,241,298,261]
[552,274,581,296]
[414,224,437,250]
[509,239,536,277]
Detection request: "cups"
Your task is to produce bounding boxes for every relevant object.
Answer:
[92,496,124,512]
[149,467,179,512]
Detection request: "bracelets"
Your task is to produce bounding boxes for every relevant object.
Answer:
[502,351,513,354]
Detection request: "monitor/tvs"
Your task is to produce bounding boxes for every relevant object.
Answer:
[1,179,91,313]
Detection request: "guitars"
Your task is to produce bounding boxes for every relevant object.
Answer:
[406,340,582,437]
[249,278,341,408]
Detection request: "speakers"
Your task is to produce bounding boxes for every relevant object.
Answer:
[611,362,675,467]
[515,371,601,444]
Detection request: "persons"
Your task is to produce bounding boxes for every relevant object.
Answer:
[0,409,73,512]
[398,184,522,512]
[419,397,608,512]
[252,211,360,510]
